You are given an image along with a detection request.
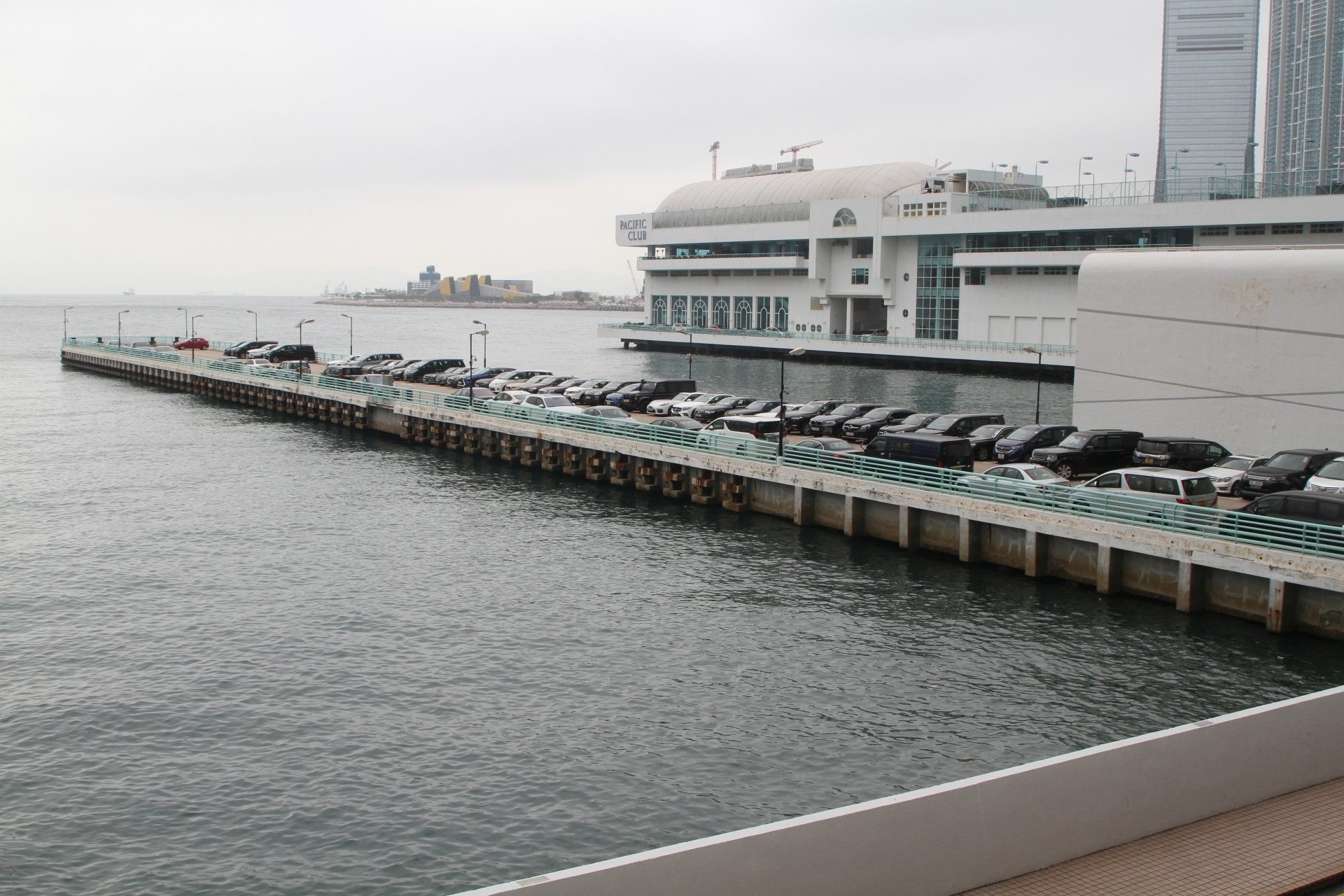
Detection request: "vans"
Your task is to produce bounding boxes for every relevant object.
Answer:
[1301,455,1344,495]
[208,340,316,377]
[992,424,1079,466]
[861,329,887,343]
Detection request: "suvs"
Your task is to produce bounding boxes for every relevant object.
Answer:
[1216,490,1344,561]
[1067,465,1218,530]
[1027,428,1144,480]
[1131,436,1234,472]
[1239,447,1344,505]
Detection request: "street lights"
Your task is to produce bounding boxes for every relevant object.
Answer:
[340,313,353,355]
[472,320,487,368]
[470,330,489,407]
[1023,345,1042,424]
[294,318,315,379]
[118,310,130,351]
[676,329,692,379]
[64,306,74,345]
[989,136,1344,213]
[246,310,257,341]
[191,314,204,363]
[178,307,188,338]
[778,347,806,457]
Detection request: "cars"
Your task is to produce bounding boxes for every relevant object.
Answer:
[672,322,792,338]
[953,462,1072,507]
[318,353,1022,560]
[1195,453,1272,498]
[173,337,210,350]
